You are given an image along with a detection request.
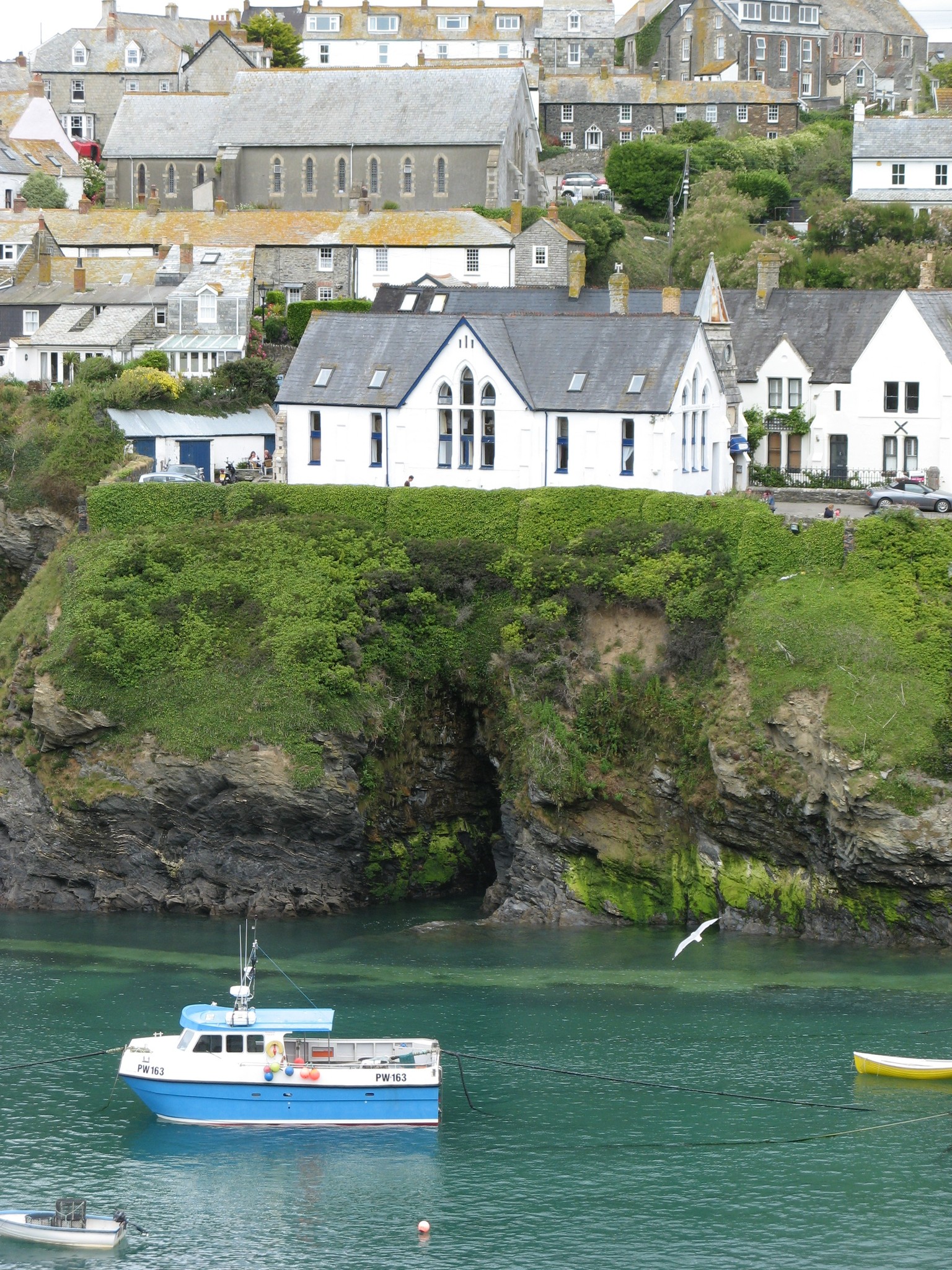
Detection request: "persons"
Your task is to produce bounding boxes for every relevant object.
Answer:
[248,451,261,469]
[263,450,272,466]
[904,472,908,478]
[824,504,834,518]
[764,489,775,513]
[405,475,414,487]
[833,508,841,518]
[747,489,752,494]
[705,490,712,495]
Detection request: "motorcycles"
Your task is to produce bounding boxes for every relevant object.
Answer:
[218,457,238,486]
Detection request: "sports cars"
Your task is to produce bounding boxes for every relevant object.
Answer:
[866,480,952,513]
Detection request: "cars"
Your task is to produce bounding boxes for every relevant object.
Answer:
[560,172,612,201]
[138,464,206,484]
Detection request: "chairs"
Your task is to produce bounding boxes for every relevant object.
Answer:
[64,380,70,387]
[249,460,263,475]
[264,459,273,476]
[29,380,46,395]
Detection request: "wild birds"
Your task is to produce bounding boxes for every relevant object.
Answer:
[672,916,722,961]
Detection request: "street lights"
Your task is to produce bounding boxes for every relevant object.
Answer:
[643,195,674,286]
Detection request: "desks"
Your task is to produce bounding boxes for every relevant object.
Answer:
[44,382,61,393]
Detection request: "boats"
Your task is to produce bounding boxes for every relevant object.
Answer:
[0,1199,150,1249]
[852,1050,952,1081]
[118,915,444,1128]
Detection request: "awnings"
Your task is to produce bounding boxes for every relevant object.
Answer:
[730,435,749,453]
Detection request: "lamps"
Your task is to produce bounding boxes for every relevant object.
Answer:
[790,522,800,533]
[25,354,28,361]
[649,415,656,423]
